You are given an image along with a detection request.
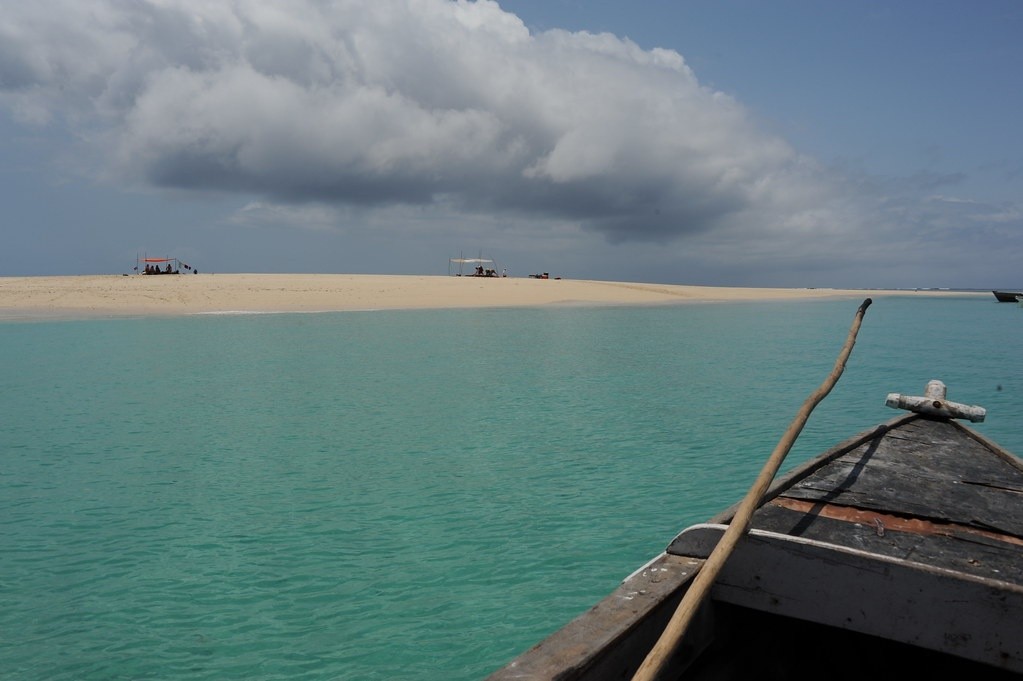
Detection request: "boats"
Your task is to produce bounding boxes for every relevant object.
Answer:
[991,290,1023,302]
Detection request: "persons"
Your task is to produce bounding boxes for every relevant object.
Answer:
[476,266,497,277]
[145,264,161,274]
[166,264,172,273]
[503,268,507,277]
